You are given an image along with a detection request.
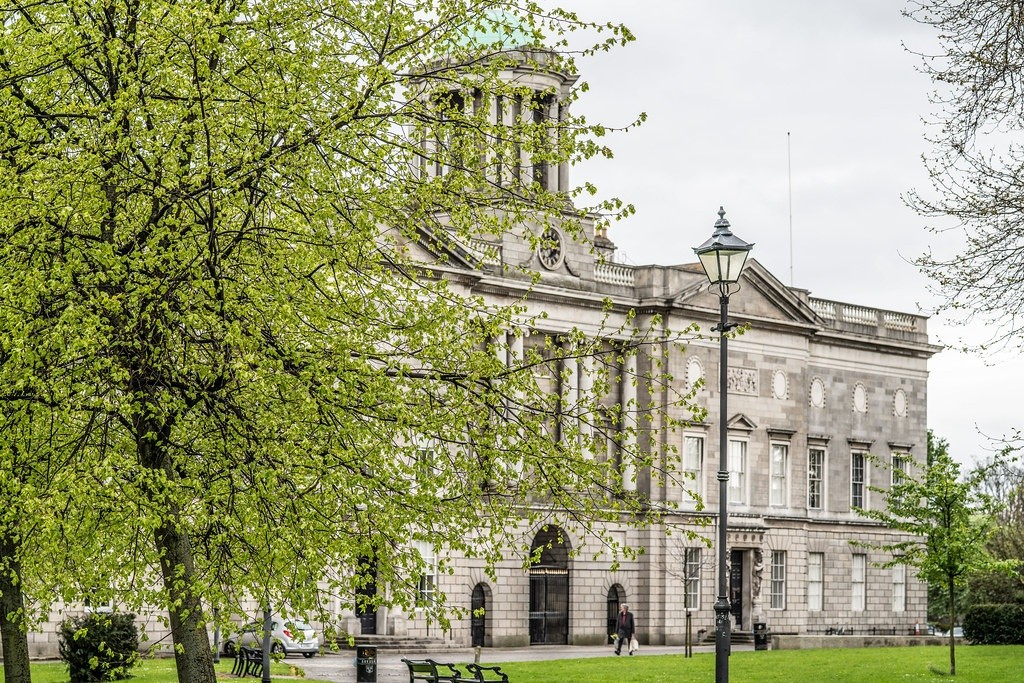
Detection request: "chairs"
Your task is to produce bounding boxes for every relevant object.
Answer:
[231,646,263,678]
[400,658,510,683]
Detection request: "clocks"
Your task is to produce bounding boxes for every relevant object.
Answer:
[539,229,561,267]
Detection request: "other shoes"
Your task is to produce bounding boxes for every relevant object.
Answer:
[615,650,620,655]
[629,652,633,655]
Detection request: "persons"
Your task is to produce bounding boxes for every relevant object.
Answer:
[614,604,635,655]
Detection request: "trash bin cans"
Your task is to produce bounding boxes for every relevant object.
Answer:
[754,623,768,650]
[61,612,137,683]
[357,645,378,683]
[927,623,935,635]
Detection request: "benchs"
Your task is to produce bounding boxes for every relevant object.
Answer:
[869,627,897,636]
[825,627,853,635]
[909,629,935,636]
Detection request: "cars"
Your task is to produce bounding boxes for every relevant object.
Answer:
[945,627,964,637]
[222,618,318,660]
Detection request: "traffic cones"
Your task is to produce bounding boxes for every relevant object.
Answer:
[915,620,920,635]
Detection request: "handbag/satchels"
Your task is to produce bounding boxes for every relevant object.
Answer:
[613,637,620,649]
[628,634,639,652]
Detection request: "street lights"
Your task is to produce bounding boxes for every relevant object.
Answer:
[691,206,755,683]
